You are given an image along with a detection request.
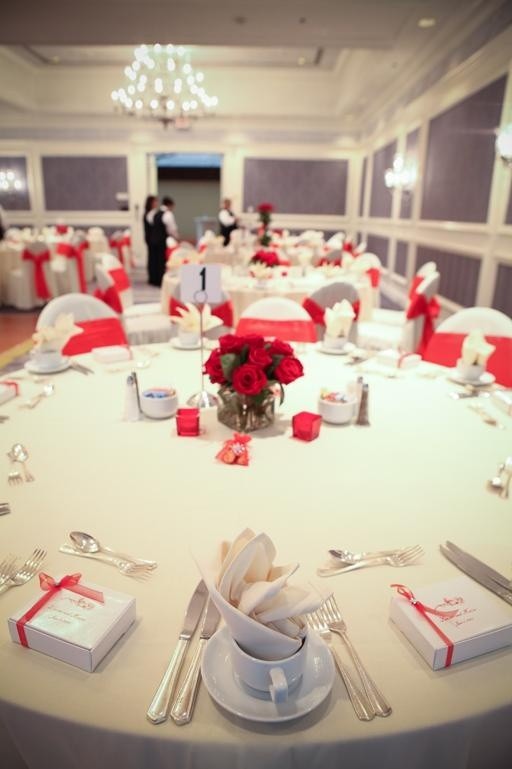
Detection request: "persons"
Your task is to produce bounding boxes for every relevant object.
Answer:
[143,196,158,245]
[146,197,180,287]
[217,198,242,246]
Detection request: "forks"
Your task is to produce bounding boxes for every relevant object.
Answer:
[304,604,373,720]
[5,447,23,487]
[0,547,50,596]
[315,543,430,582]
[60,542,147,579]
[323,592,396,720]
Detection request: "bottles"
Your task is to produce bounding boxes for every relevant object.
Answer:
[352,376,372,426]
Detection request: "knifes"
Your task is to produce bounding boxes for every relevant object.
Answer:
[439,539,511,606]
[140,578,210,724]
[126,372,141,412]
[169,585,226,725]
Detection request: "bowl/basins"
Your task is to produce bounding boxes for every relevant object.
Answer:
[318,392,358,427]
[139,385,179,419]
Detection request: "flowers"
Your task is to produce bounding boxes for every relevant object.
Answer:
[201,330,307,432]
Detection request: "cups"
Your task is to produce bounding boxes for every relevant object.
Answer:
[321,332,347,348]
[291,411,323,441]
[179,327,199,344]
[228,630,310,707]
[175,408,201,437]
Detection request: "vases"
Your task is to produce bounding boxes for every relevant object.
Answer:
[215,380,277,434]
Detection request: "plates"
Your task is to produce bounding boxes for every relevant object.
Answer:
[200,626,338,724]
[311,342,501,387]
[22,338,211,376]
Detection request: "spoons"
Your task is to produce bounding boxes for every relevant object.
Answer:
[326,547,393,564]
[70,532,161,570]
[12,441,37,484]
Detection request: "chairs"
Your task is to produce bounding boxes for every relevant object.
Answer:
[421,307,512,384]
[0,221,138,311]
[380,259,440,353]
[164,228,380,341]
[33,292,130,385]
[93,251,165,347]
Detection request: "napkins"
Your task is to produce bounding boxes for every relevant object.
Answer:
[33,313,84,366]
[455,327,496,381]
